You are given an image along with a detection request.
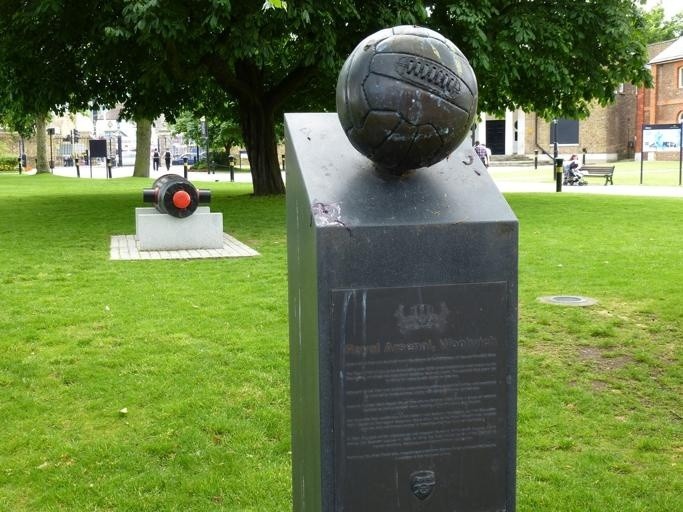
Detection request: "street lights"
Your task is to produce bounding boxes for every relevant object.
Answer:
[552,116,560,182]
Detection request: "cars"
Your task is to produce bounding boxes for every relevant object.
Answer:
[170,151,236,168]
[115,150,136,166]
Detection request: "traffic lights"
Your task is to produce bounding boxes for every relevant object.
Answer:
[73,129,78,143]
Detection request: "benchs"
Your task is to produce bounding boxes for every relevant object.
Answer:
[576,163,616,188]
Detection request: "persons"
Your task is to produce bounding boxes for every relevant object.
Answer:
[152,148,161,171]
[564,155,578,180]
[474,140,489,170]
[161,148,172,172]
[479,144,493,168]
